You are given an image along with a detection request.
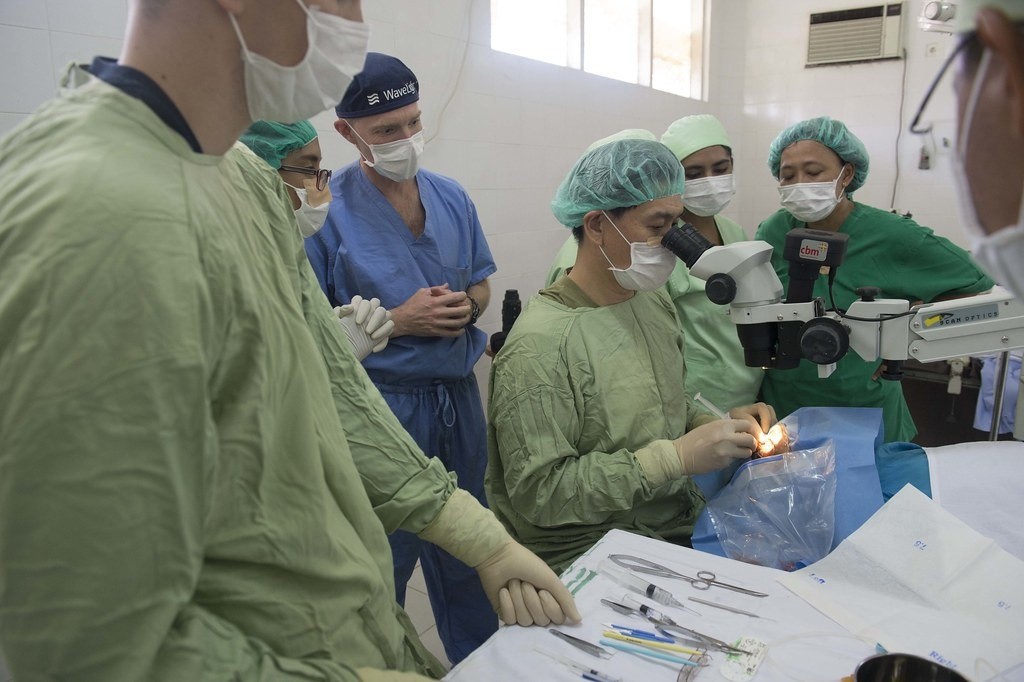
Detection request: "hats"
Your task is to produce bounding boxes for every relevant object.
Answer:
[335,53,420,118]
[661,115,733,162]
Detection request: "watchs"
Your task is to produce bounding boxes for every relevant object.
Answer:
[467,295,480,325]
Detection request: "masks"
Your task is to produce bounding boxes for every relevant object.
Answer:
[283,180,329,237]
[946,48,1024,301]
[598,210,677,292]
[343,120,425,182]
[683,174,736,217]
[778,166,846,223]
[228,1,369,124]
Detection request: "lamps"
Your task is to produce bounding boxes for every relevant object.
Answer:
[924,0,955,22]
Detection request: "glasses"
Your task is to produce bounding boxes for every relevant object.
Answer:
[909,33,977,154]
[281,164,332,191]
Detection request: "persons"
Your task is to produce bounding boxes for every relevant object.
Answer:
[239,119,395,361]
[926,0,1024,301]
[548,115,765,510]
[0,0,581,682]
[487,137,780,576]
[755,115,991,447]
[301,52,499,668]
